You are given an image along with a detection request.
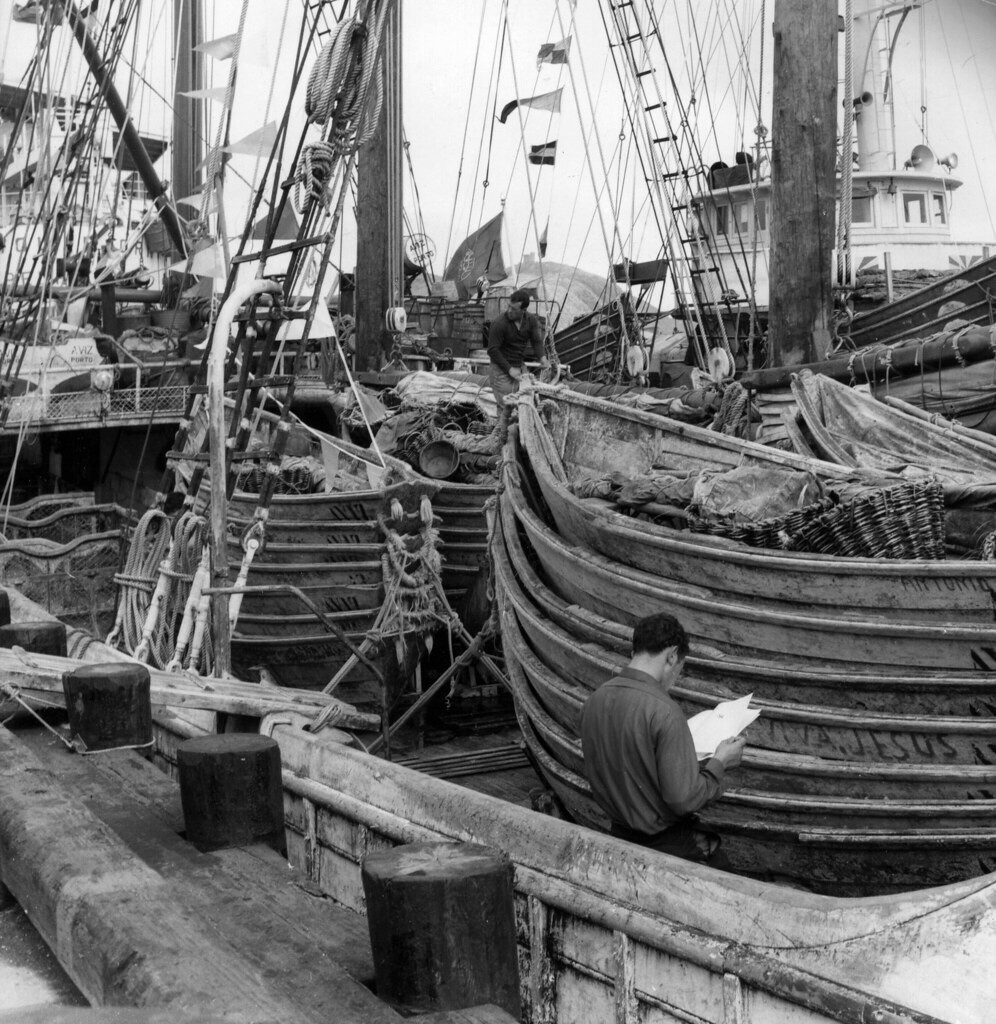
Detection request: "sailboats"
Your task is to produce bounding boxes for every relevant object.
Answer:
[3,0,996,1024]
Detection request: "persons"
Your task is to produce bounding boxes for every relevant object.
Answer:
[486,289,553,422]
[578,612,746,863]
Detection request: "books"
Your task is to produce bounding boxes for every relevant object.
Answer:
[685,692,760,762]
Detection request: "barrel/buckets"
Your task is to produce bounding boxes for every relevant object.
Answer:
[404,301,432,331]
[453,305,485,350]
[419,440,460,478]
[115,314,151,337]
[270,422,312,457]
[152,310,190,332]
[430,301,456,337]
